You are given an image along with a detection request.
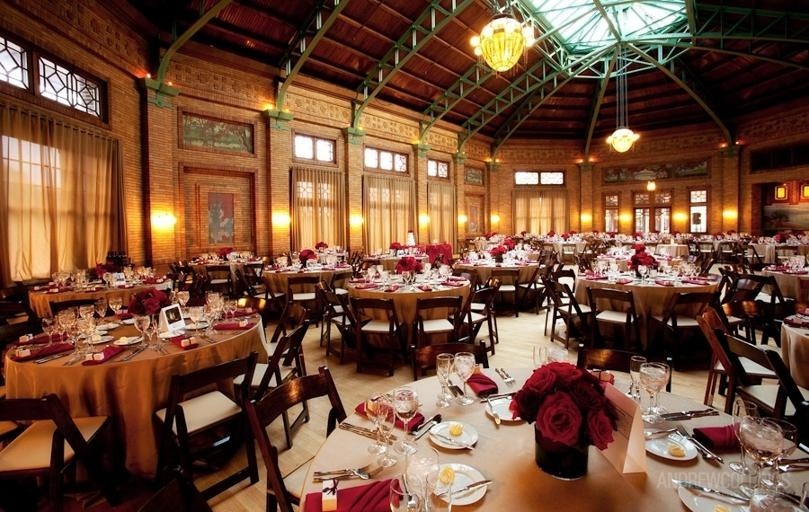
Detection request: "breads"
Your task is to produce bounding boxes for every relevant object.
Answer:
[439,465,456,482]
[120,336,127,342]
[714,504,729,512]
[668,445,686,456]
[449,425,464,435]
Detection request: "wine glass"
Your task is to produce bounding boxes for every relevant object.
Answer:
[266,227,795,302]
[313,343,809,511]
[15,248,256,365]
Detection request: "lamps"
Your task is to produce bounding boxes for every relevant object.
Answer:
[605,42,641,153]
[480,0,525,73]
